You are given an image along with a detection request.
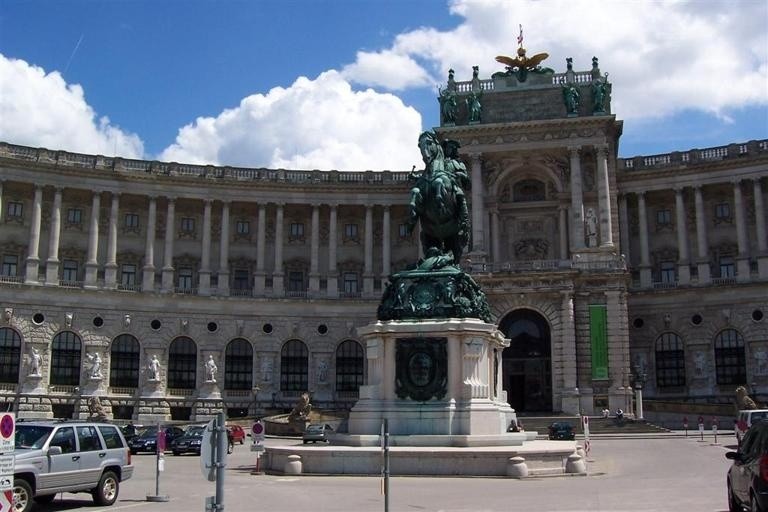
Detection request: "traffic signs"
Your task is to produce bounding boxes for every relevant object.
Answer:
[0,491,13,511]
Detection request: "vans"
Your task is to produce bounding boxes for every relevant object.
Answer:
[735,409,767,444]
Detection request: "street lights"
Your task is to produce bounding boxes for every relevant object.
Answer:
[252,384,261,421]
[633,375,644,416]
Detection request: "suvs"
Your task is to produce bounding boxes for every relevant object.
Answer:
[12,417,134,512]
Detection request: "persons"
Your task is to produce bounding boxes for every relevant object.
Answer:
[438,72,612,129]
[403,139,477,244]
[602,406,624,418]
[29,345,219,381]
[125,420,136,435]
[507,418,520,432]
[157,426,166,452]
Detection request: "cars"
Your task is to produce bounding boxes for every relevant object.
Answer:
[302,423,334,443]
[548,422,576,439]
[128,425,183,454]
[172,426,234,454]
[227,425,245,444]
[725,416,767,510]
